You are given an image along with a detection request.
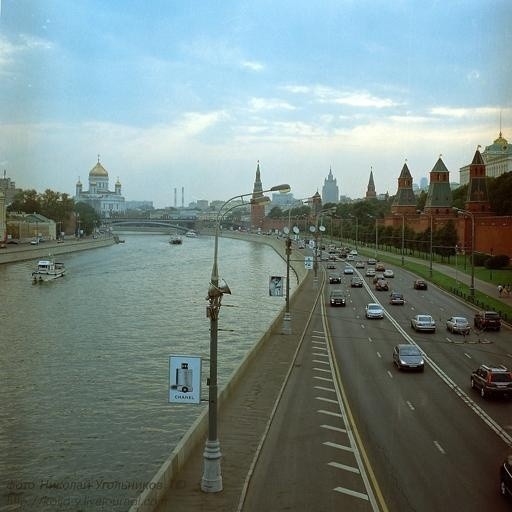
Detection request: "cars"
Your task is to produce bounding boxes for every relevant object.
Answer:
[413,280,427,290]
[31,239,37,245]
[39,239,45,242]
[364,303,384,319]
[330,289,346,306]
[410,314,436,333]
[94,226,114,239]
[445,316,471,335]
[389,292,405,305]
[392,344,424,371]
[322,247,394,292]
[221,224,263,234]
[57,240,65,244]
[500,455,512,502]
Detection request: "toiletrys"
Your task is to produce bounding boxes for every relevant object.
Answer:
[170,361,194,392]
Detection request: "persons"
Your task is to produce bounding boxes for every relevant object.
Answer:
[497,283,511,297]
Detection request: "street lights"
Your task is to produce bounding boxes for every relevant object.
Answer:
[334,213,344,247]
[368,213,379,258]
[390,209,406,266]
[416,209,434,278]
[201,184,292,493]
[263,207,337,290]
[349,214,359,251]
[273,195,321,334]
[452,206,478,299]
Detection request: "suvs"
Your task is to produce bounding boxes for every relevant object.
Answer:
[470,364,512,400]
[473,311,502,332]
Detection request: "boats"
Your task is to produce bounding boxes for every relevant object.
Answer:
[169,233,184,245]
[32,258,65,284]
[186,229,197,239]
